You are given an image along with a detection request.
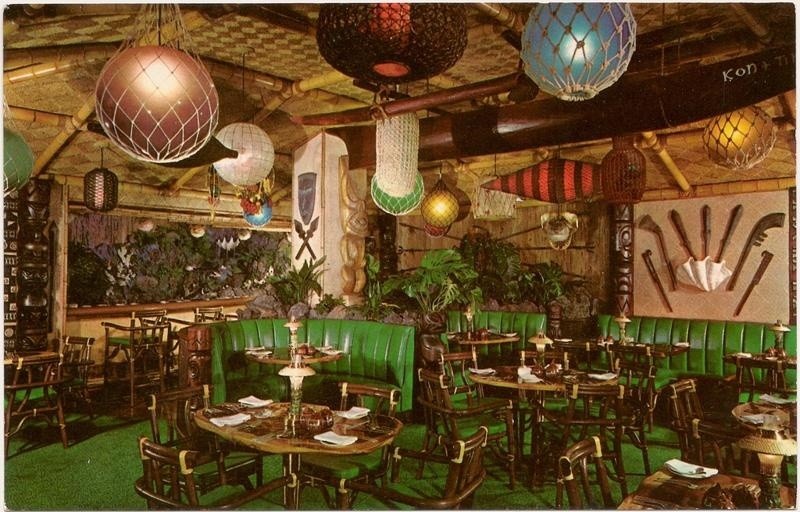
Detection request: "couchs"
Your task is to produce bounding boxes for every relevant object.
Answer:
[444,311,547,355]
[177,318,420,425]
[573,316,796,412]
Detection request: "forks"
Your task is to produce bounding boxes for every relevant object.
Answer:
[653,476,699,490]
[632,494,680,509]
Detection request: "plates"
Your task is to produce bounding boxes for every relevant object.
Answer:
[666,461,720,481]
[212,395,370,449]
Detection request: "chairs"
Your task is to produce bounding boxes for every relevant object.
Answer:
[6,315,172,456]
[135,332,796,509]
